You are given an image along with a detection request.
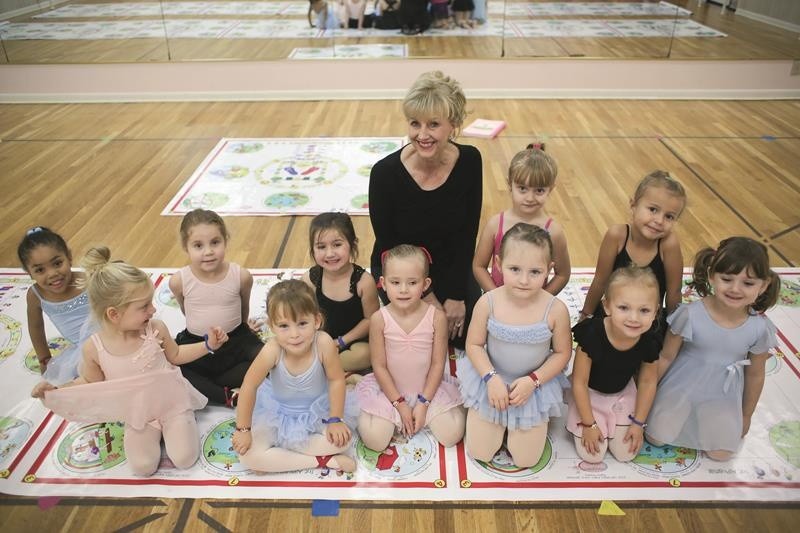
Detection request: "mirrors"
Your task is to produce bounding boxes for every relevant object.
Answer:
[0,0,800,66]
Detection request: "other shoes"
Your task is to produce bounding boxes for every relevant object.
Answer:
[345,372,361,383]
[401,22,409,35]
[224,387,239,406]
[408,26,422,35]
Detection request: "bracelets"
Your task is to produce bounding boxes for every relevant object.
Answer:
[236,427,251,432]
[627,414,648,429]
[484,370,498,383]
[391,396,405,406]
[576,420,597,430]
[322,416,345,424]
[417,393,431,405]
[204,333,216,355]
[338,336,349,348]
[579,310,594,319]
[55,386,57,389]
[529,372,540,387]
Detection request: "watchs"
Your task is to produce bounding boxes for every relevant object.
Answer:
[17,71,781,477]
[39,356,51,365]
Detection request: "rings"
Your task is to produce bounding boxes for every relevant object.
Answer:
[454,323,460,328]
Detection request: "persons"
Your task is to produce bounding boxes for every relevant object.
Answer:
[307,0,488,30]
